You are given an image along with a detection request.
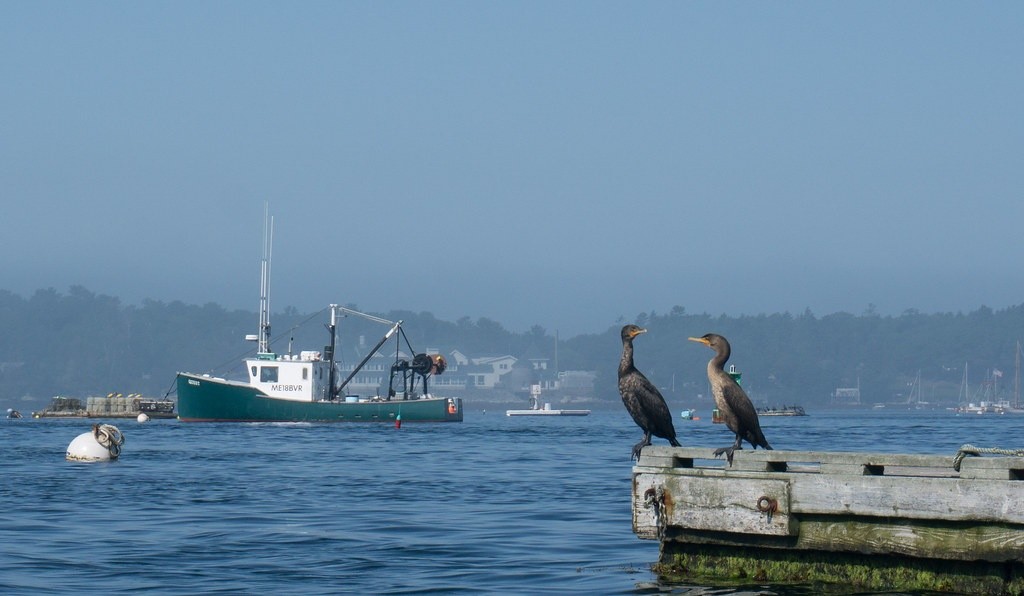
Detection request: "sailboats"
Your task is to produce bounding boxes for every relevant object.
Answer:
[903,338,1024,415]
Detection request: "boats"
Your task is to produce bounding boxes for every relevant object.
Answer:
[870,403,886,411]
[171,203,465,426]
[506,385,592,415]
[754,406,806,416]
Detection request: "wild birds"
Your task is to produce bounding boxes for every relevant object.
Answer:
[687,331,774,469]
[617,323,684,463]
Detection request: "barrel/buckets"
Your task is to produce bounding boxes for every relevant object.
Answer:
[346,395,359,403]
